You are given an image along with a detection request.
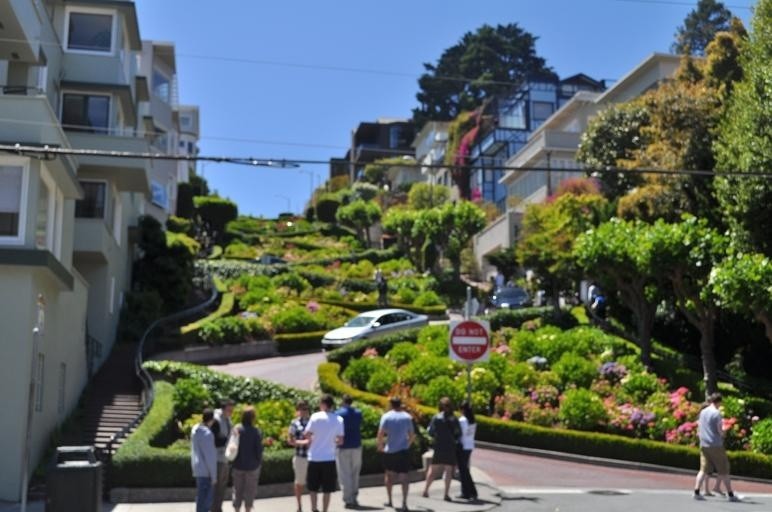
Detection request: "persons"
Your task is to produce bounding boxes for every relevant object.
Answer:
[421,396,462,502]
[455,400,478,499]
[224,406,264,512]
[376,396,415,511]
[497,268,608,319]
[693,391,742,503]
[210,398,237,512]
[331,394,366,510]
[287,399,312,512]
[190,408,218,511]
[697,396,726,497]
[373,267,389,309]
[302,394,345,512]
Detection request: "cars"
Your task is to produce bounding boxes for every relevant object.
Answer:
[317,302,427,359]
[482,282,534,317]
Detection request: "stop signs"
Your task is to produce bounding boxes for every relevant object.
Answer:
[448,320,491,362]
